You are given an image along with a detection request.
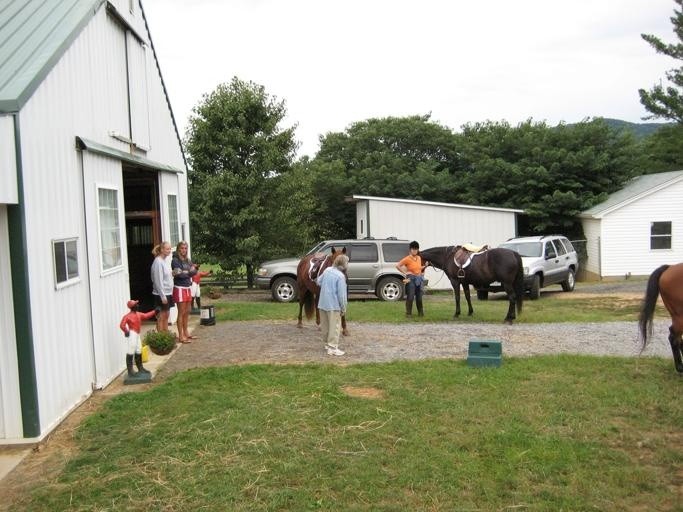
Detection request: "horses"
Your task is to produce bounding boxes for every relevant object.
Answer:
[636,262,683,377]
[296,246,349,336]
[417,245,524,325]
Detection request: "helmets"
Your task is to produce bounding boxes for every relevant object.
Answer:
[410,241,418,247]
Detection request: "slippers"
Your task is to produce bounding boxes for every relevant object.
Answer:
[179,335,197,343]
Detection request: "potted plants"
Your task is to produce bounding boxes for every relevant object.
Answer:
[144,328,178,356]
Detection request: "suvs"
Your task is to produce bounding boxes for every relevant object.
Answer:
[475,233,578,298]
[254,235,426,302]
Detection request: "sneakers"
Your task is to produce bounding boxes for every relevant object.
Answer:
[328,348,345,355]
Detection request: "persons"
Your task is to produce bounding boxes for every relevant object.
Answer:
[150,241,175,333]
[188,263,214,310]
[313,254,353,357]
[395,240,429,318]
[172,240,200,344]
[119,300,161,378]
